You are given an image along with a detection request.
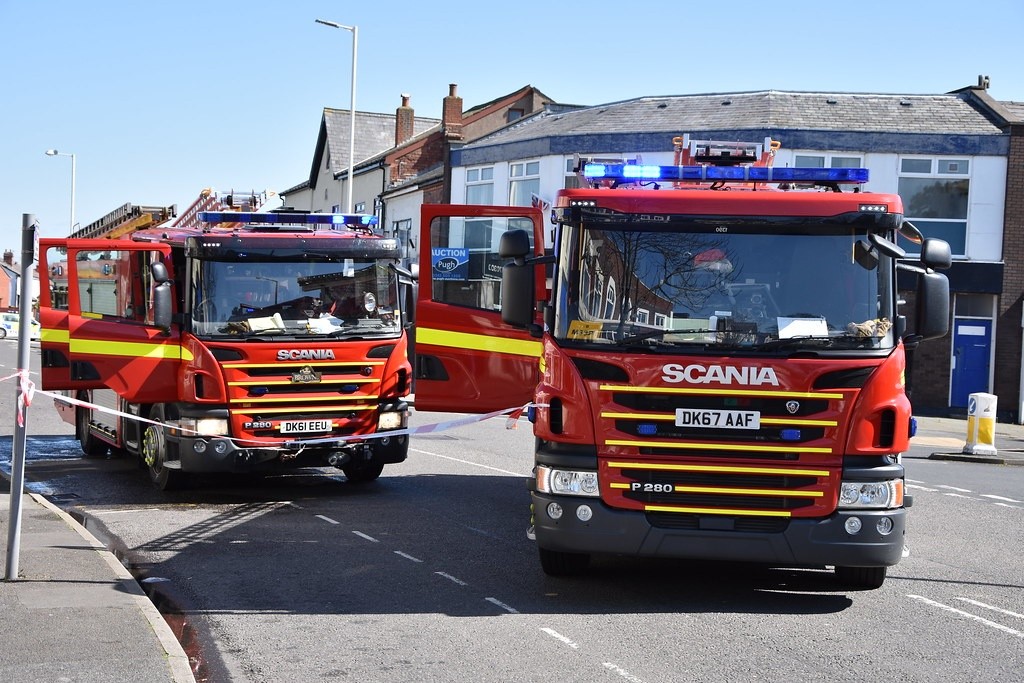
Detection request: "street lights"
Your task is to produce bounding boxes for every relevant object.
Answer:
[315,18,358,214]
[46,149,76,237]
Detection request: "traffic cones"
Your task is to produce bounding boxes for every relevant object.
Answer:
[962,392,998,456]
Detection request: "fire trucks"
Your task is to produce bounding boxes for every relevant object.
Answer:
[413,131,954,591]
[37,209,410,493]
